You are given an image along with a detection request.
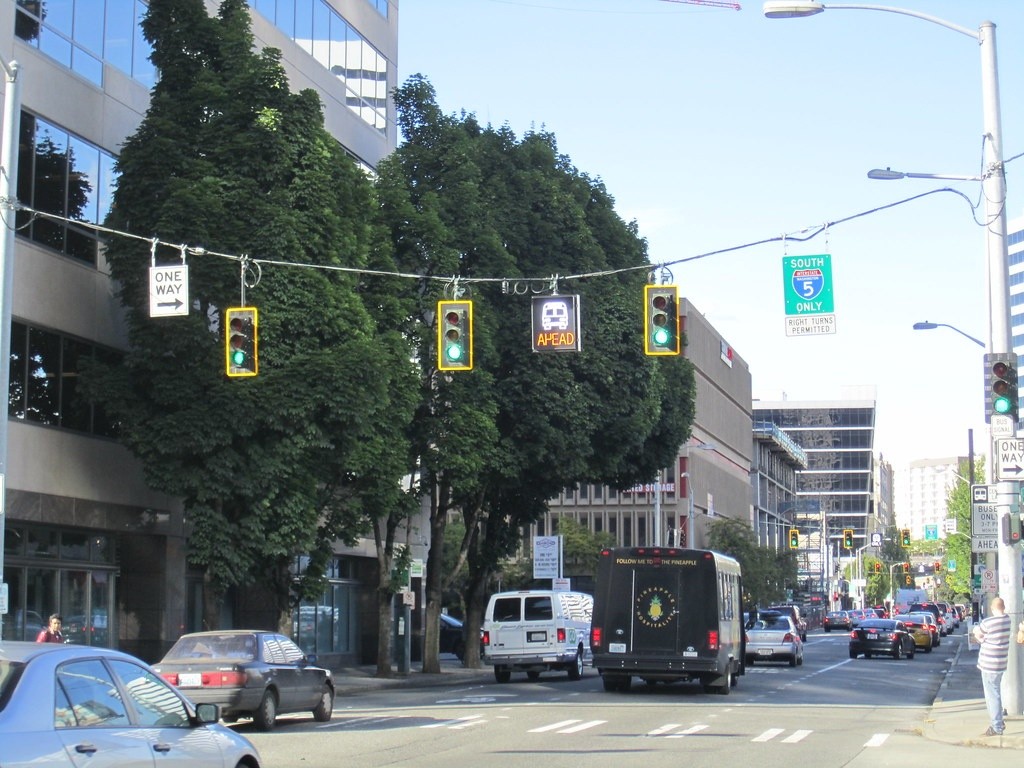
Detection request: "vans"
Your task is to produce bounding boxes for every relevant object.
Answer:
[481,545,749,697]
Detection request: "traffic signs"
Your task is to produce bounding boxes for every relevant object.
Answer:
[150,266,188,319]
[996,437,1024,480]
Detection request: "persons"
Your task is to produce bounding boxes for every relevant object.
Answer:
[37,614,64,643]
[973,597,1012,737]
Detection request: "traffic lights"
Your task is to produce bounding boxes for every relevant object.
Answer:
[789,530,799,548]
[1002,513,1021,545]
[645,285,680,355]
[935,563,939,572]
[903,564,909,574]
[437,301,473,370]
[844,530,853,549]
[225,308,257,377]
[983,352,1019,425]
[901,529,911,548]
[875,563,880,573]
[906,576,911,585]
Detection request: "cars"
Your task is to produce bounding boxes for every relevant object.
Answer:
[0,641,263,768]
[147,598,971,734]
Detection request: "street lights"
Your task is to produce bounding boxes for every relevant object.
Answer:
[652,443,716,548]
[681,472,695,549]
[762,3,1024,716]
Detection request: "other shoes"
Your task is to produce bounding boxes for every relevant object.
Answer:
[985,722,1007,736]
